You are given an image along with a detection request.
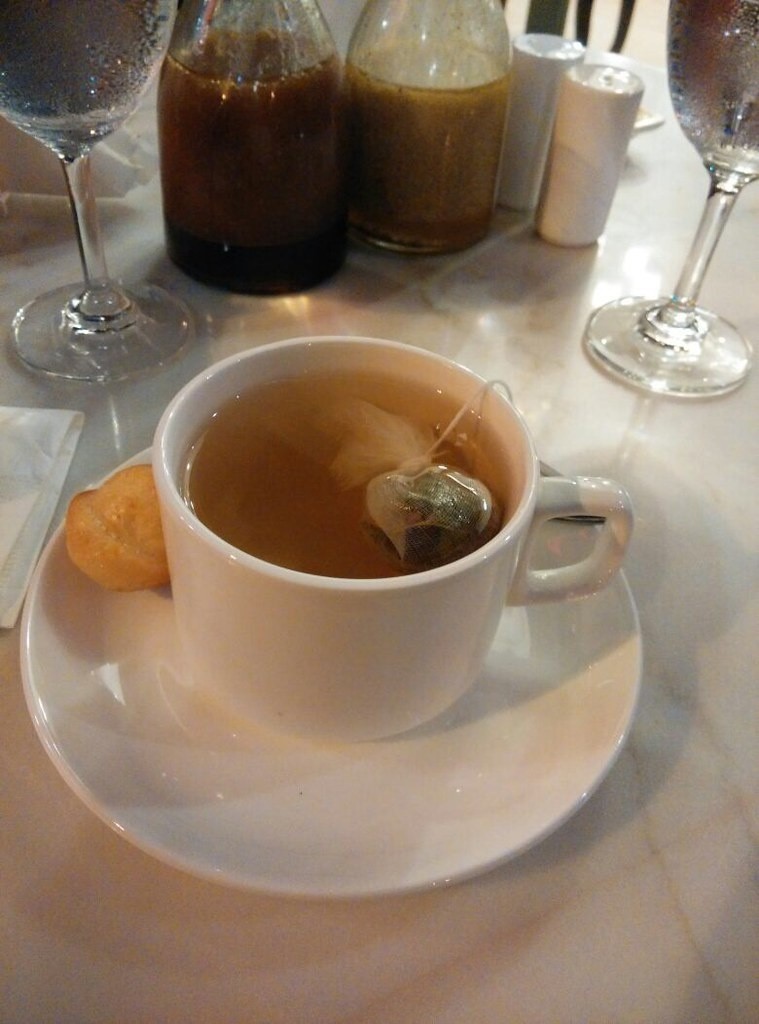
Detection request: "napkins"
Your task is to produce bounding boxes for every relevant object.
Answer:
[0,405,84,628]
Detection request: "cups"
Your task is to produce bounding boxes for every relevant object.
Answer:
[152,336,633,744]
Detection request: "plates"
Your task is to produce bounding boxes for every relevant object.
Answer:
[20,447,642,897]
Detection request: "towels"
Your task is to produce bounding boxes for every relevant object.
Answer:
[0,405,85,629]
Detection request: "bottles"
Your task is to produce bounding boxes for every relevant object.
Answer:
[344,0,511,253]
[158,0,350,296]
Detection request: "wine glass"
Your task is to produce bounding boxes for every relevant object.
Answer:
[0,0,194,381]
[585,0,759,398]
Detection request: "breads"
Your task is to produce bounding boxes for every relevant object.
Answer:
[63,464,170,594]
